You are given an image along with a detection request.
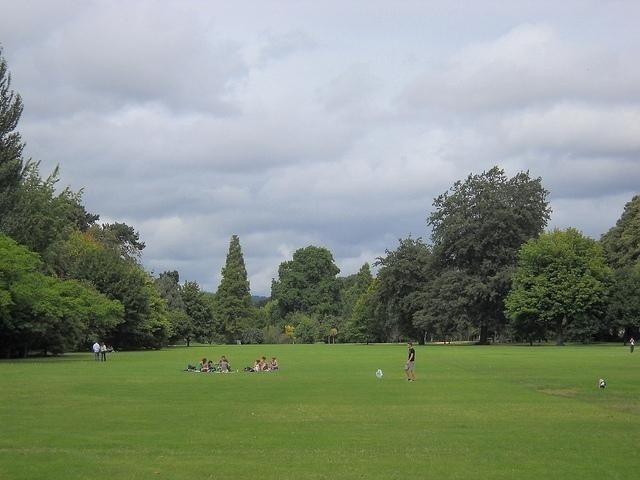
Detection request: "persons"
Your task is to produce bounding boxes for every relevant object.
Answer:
[245,356,279,372]
[184,355,231,373]
[93,340,100,361]
[100,342,107,362]
[105,344,115,352]
[404,342,416,382]
[629,337,635,353]
